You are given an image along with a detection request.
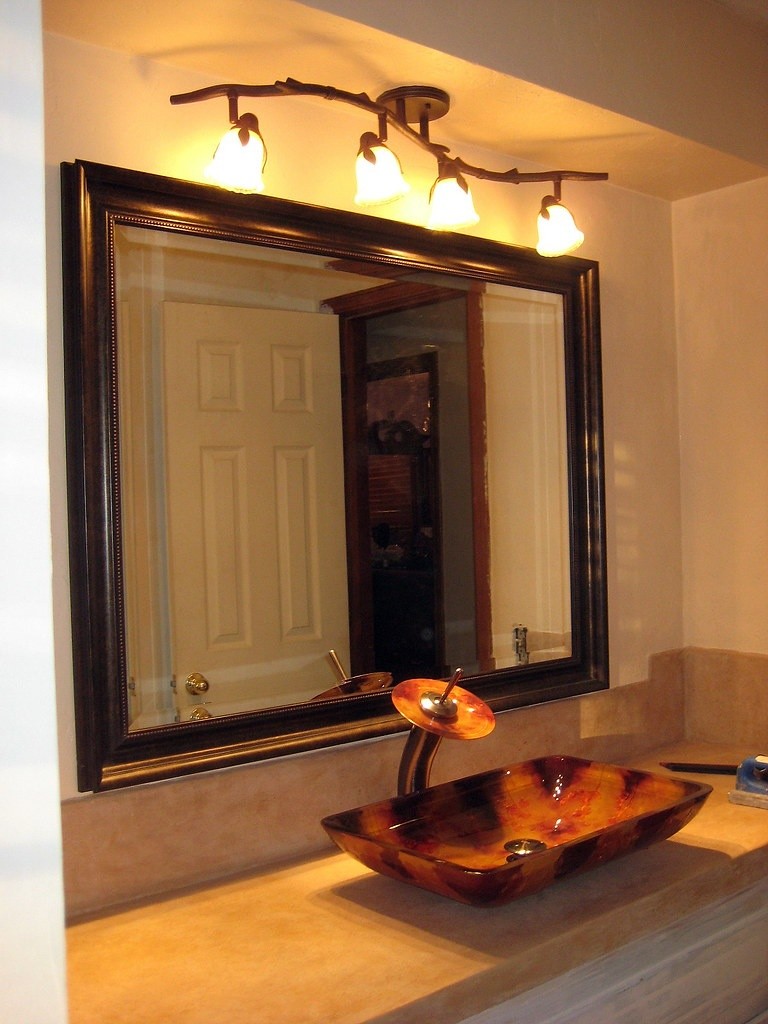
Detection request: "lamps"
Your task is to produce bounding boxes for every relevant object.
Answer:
[171,78,608,259]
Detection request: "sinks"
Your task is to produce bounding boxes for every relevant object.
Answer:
[322,756,714,908]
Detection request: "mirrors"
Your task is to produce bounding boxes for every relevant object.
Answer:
[71,158,612,792]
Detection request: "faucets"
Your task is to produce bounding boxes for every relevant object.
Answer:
[391,668,497,795]
[309,650,391,702]
